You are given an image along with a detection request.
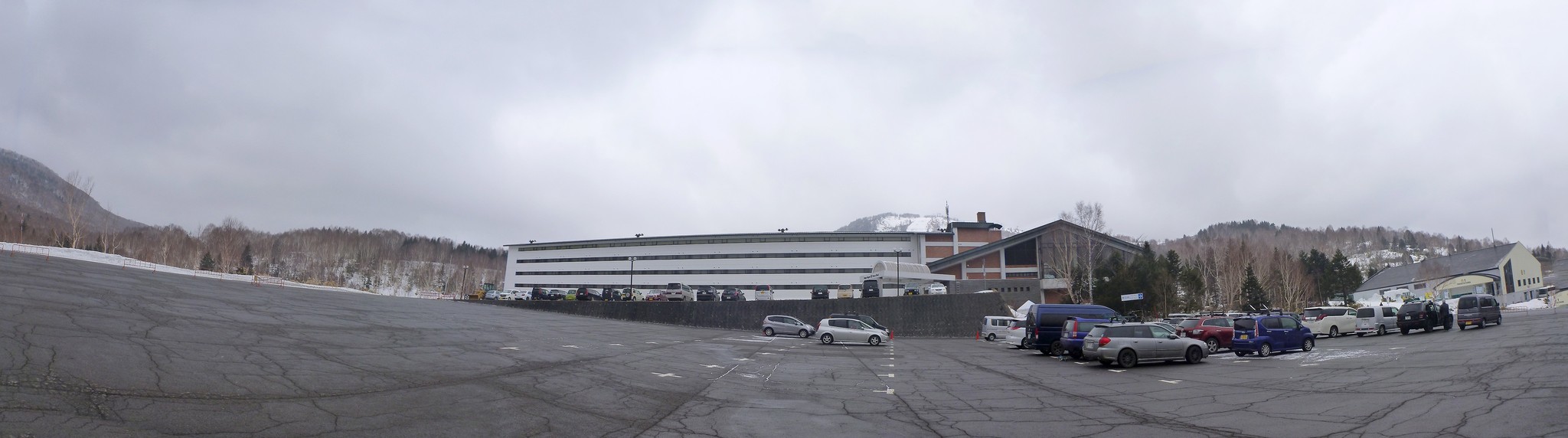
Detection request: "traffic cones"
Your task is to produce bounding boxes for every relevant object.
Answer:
[975,330,980,339]
[889,330,894,339]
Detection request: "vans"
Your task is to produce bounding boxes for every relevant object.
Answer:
[858,279,879,298]
[1301,306,1358,338]
[1354,305,1400,338]
[828,310,890,336]
[1024,304,1128,357]
[981,315,1015,341]
[1457,293,1502,330]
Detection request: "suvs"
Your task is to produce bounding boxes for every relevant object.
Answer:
[903,282,921,295]
[1396,300,1454,335]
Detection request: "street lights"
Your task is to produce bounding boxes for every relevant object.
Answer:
[628,256,637,300]
[460,265,469,300]
[893,248,903,296]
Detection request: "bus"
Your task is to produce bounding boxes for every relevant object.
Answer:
[1537,285,1556,298]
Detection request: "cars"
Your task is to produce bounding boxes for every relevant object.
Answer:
[1082,312,1210,368]
[815,318,889,346]
[468,282,775,302]
[837,284,854,298]
[762,315,816,338]
[928,283,947,294]
[1059,316,1121,362]
[810,285,830,299]
[1005,318,1033,350]
[1161,307,1315,357]
